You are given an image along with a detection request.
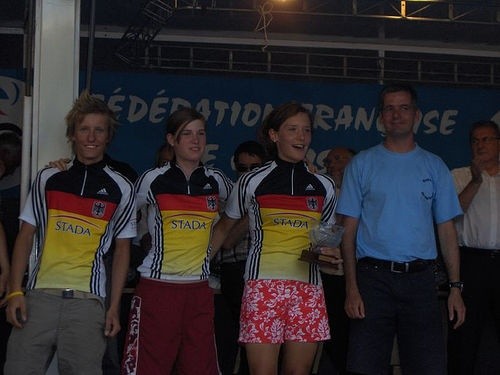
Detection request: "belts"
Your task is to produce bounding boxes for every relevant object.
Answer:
[30,287,105,310]
[358,255,434,273]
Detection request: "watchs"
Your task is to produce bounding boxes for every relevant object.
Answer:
[450,281,464,290]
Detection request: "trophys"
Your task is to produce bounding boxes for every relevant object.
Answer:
[297,220,346,270]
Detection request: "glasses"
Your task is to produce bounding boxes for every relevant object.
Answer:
[471,137,499,144]
[237,165,260,172]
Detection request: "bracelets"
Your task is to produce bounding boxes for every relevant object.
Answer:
[6,290,24,299]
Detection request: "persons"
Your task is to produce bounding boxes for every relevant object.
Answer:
[446,120,500,375]
[0,222,10,308]
[44,108,320,375]
[4,91,138,375]
[208,102,337,375]
[213,139,267,375]
[321,147,363,375]
[335,80,467,375]
[132,141,175,266]
